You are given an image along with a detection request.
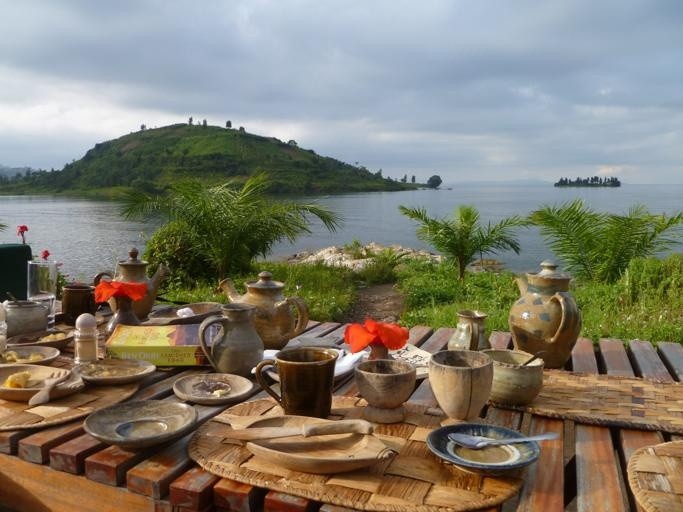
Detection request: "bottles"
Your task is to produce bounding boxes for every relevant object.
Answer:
[96,328,106,359]
[71,312,98,367]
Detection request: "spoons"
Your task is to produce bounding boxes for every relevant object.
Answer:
[446,429,562,452]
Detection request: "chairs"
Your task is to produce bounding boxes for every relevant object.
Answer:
[0,244,38,301]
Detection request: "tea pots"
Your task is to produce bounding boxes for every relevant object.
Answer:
[445,308,492,372]
[218,271,307,352]
[196,302,264,384]
[505,259,582,369]
[94,248,167,322]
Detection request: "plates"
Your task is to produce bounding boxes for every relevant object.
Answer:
[0,363,70,404]
[80,357,156,386]
[423,421,540,477]
[171,372,253,406]
[264,345,368,387]
[5,327,73,350]
[228,413,398,473]
[81,397,199,453]
[0,346,61,365]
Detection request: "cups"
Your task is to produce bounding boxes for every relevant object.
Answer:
[25,257,57,331]
[256,347,339,418]
[481,347,545,410]
[61,285,97,325]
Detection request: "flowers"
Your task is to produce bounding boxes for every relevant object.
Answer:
[343,318,410,352]
[92,281,146,304]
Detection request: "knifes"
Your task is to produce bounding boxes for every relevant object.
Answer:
[205,419,372,446]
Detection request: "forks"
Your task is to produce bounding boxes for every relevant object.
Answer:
[26,370,70,409]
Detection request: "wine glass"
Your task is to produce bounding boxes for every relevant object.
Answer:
[427,349,493,429]
[351,359,417,425]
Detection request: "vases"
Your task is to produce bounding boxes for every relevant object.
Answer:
[367,343,388,360]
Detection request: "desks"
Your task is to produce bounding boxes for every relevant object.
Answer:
[0,287,683,512]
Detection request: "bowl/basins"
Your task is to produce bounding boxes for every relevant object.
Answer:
[147,301,223,323]
[4,301,48,342]
